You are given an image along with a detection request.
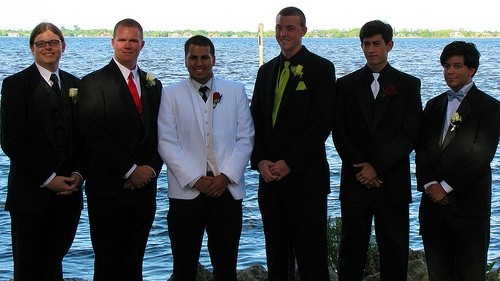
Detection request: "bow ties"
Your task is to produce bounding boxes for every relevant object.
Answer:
[445,89,467,102]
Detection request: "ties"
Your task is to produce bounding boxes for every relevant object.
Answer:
[199,86,209,103]
[271,61,290,126]
[50,74,61,96]
[128,73,142,115]
[370,73,380,98]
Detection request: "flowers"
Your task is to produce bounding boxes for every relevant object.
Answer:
[146,71,156,88]
[69,88,79,104]
[291,65,304,78]
[213,92,223,109]
[381,84,399,100]
[450,113,462,131]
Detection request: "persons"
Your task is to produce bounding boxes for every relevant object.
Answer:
[1,23,85,281]
[76,19,163,281]
[414,41,500,281]
[249,6,336,281]
[157,35,255,281]
[332,20,423,281]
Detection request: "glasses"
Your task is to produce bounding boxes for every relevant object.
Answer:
[32,39,60,48]
